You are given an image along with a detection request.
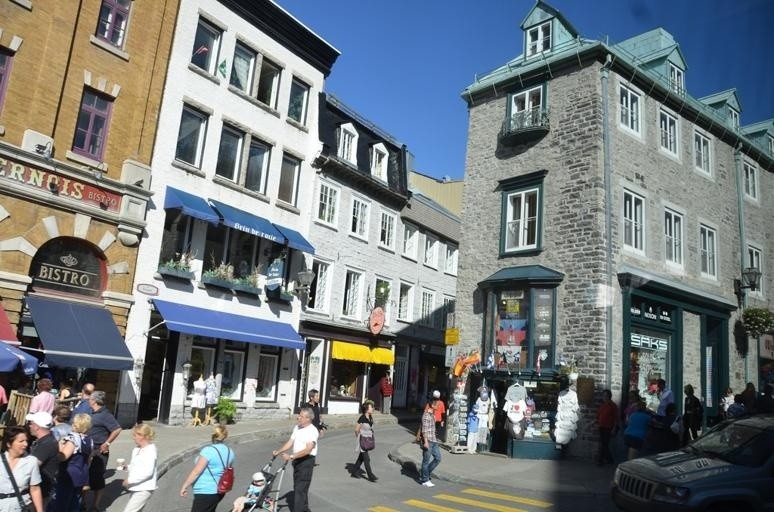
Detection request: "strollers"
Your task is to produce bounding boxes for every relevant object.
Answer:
[229,452,288,512]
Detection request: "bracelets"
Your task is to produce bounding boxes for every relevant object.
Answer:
[290,455,294,461]
[105,441,111,446]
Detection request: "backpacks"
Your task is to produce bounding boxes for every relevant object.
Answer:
[202,444,234,494]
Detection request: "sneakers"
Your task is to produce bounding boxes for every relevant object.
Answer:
[422,480,435,487]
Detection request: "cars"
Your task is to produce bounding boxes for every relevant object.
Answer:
[611,415,774,511]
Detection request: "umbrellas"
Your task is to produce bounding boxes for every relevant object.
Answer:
[0,340,39,376]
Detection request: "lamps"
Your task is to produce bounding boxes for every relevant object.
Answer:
[182,357,193,380]
[292,262,316,291]
[93,161,104,180]
[42,141,52,161]
[734,266,761,295]
[134,356,143,385]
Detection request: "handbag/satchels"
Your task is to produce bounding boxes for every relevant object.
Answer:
[670,422,681,435]
[359,436,376,450]
[644,416,664,442]
[21,503,37,512]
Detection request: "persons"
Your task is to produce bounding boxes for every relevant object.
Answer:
[656,378,675,415]
[757,383,774,414]
[69,383,94,423]
[87,391,121,510]
[179,424,235,512]
[682,383,704,440]
[272,407,319,511]
[596,389,619,464]
[0,381,8,412]
[1,427,44,512]
[720,386,733,418]
[648,402,680,456]
[231,471,266,512]
[57,372,77,399]
[429,389,444,437]
[301,388,322,458]
[726,393,744,418]
[741,383,762,415]
[27,378,55,415]
[120,423,158,511]
[25,411,60,512]
[57,413,93,511]
[622,403,657,459]
[351,399,377,481]
[415,396,442,488]
[49,404,72,442]
[207,423,208,425]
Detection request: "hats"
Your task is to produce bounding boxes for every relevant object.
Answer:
[433,390,440,398]
[251,472,266,482]
[25,412,54,429]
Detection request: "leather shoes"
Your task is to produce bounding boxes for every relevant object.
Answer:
[351,473,360,478]
[368,476,378,482]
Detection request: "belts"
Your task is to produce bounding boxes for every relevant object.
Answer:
[0,489,30,499]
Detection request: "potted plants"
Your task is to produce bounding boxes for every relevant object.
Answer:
[212,396,236,425]
[158,246,200,280]
[282,290,294,302]
[234,263,263,295]
[202,261,234,289]
